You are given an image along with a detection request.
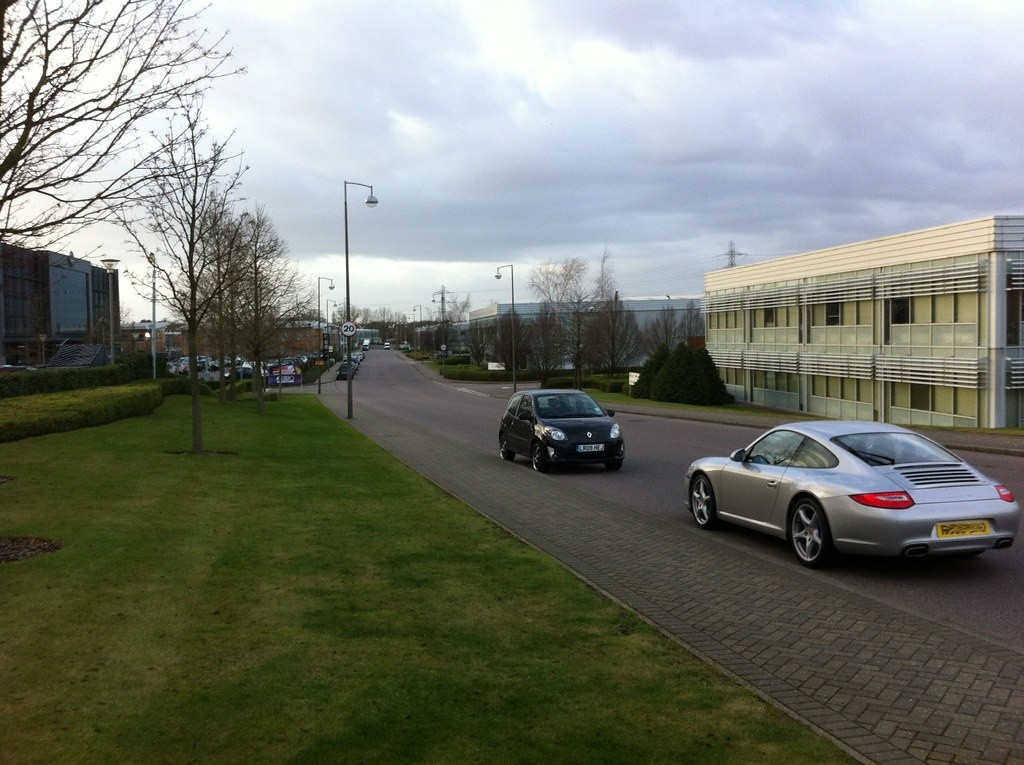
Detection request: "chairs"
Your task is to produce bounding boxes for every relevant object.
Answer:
[549,398,562,417]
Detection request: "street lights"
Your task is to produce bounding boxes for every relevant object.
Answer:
[495,263,516,396]
[342,180,378,419]
[431,292,449,378]
[413,305,424,362]
[100,258,121,364]
[318,276,337,354]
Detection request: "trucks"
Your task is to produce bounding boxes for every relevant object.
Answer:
[361,338,371,352]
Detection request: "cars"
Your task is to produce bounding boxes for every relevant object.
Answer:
[497,388,626,474]
[684,420,1020,570]
[165,353,319,379]
[335,352,365,380]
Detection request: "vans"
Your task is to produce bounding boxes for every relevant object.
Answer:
[384,342,390,349]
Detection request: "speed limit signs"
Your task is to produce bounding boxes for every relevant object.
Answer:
[341,321,357,337]
